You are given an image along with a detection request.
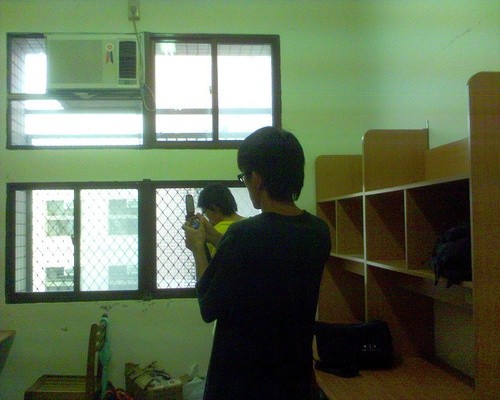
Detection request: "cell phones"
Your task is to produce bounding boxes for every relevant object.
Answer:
[185,194,200,230]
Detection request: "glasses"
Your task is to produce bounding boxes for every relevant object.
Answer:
[238,172,254,182]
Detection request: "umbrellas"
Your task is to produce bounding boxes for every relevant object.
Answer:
[97,317,114,400]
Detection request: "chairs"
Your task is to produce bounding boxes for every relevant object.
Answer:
[24,312,110,400]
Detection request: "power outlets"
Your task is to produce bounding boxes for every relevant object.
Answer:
[126,1,140,21]
[128,0,141,19]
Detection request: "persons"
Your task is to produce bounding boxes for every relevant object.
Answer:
[182,126,332,400]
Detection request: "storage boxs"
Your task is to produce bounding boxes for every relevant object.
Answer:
[124,362,183,400]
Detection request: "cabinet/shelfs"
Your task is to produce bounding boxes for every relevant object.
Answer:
[310,72,500,400]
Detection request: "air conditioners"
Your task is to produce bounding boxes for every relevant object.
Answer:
[44,33,148,100]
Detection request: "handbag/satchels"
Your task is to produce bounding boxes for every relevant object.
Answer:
[314,320,394,379]
[431,222,472,290]
[180,364,205,400]
